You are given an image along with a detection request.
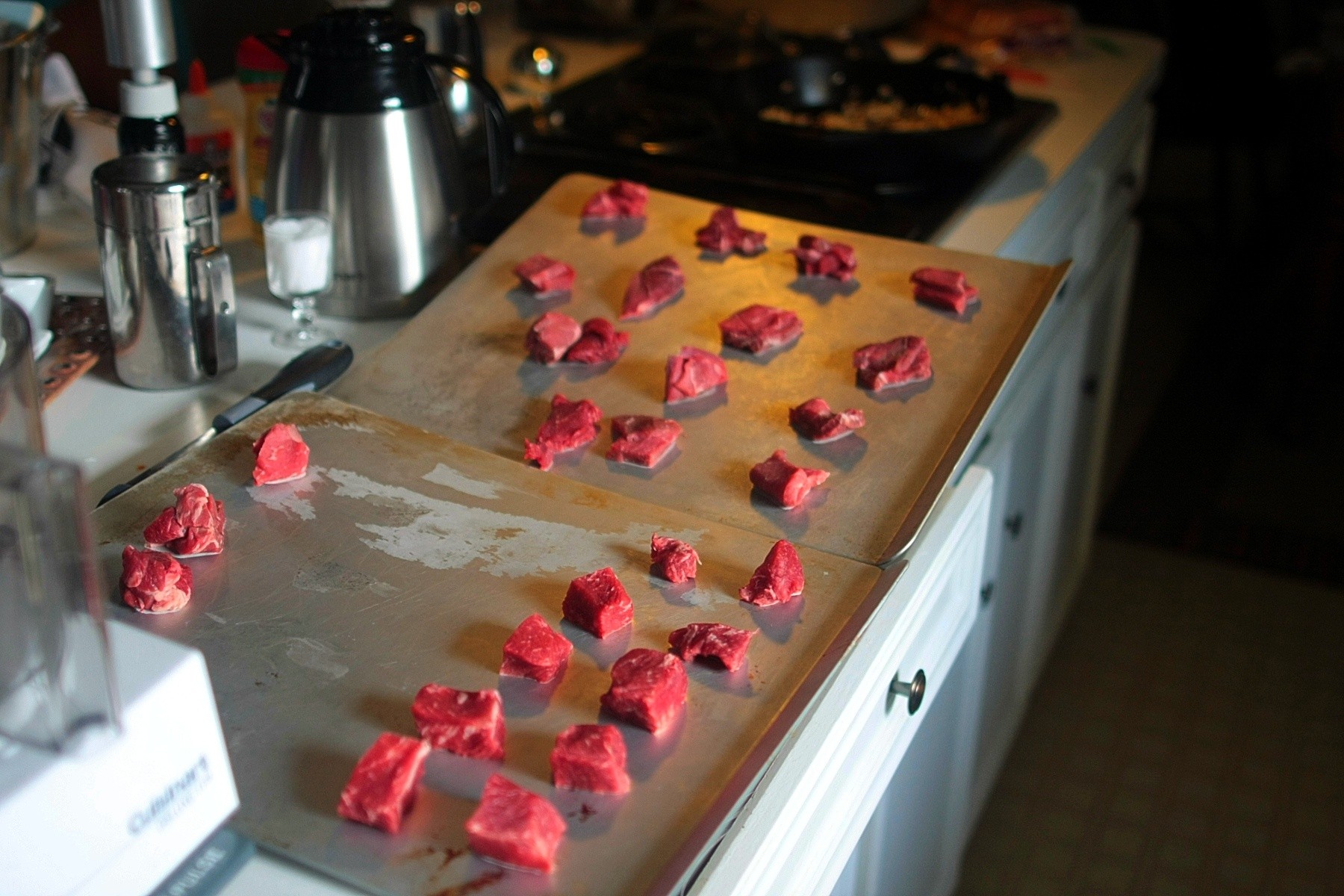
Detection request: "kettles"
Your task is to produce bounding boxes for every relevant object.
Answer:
[249,10,514,320]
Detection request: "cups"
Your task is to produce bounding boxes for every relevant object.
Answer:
[1,292,120,756]
[0,12,56,262]
[90,151,237,390]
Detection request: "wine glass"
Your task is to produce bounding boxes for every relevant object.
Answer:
[261,208,335,351]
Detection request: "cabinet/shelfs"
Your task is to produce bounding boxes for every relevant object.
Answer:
[667,100,1151,896]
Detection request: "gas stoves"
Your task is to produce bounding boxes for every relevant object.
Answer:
[454,29,1058,268]
